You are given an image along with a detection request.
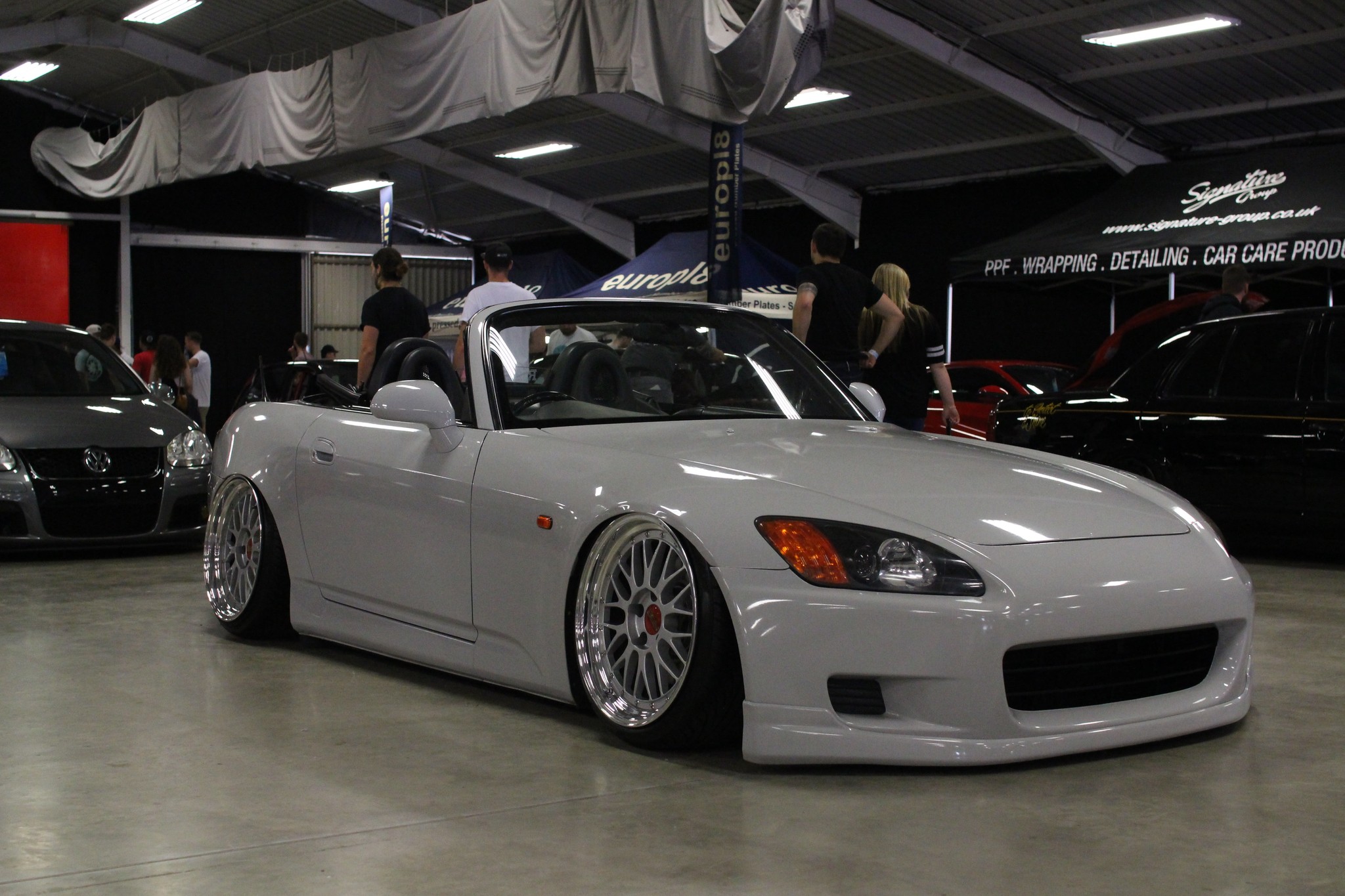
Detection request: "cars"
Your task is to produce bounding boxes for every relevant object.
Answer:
[988,297,1344,568]
[919,356,1084,445]
[0,316,213,559]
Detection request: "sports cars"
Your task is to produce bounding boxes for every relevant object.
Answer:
[199,294,1254,781]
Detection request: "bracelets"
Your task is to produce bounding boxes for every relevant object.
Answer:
[868,349,878,359]
[944,406,956,409]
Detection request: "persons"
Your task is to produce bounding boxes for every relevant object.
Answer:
[149,334,192,395]
[74,324,101,375]
[320,345,339,360]
[357,248,431,394]
[1199,265,1265,321]
[132,333,157,382]
[451,241,545,383]
[621,322,724,403]
[791,224,905,379]
[84,324,122,395]
[288,333,315,360]
[546,322,598,356]
[184,332,212,430]
[599,324,633,350]
[859,263,960,432]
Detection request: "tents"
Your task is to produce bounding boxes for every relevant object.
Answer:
[423,251,600,358]
[944,142,1345,362]
[557,229,800,319]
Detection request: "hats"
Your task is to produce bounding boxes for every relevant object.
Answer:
[481,242,513,260]
[321,344,339,353]
[86,324,100,335]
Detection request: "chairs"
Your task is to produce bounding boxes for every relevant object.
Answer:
[363,338,470,421]
[541,341,638,411]
